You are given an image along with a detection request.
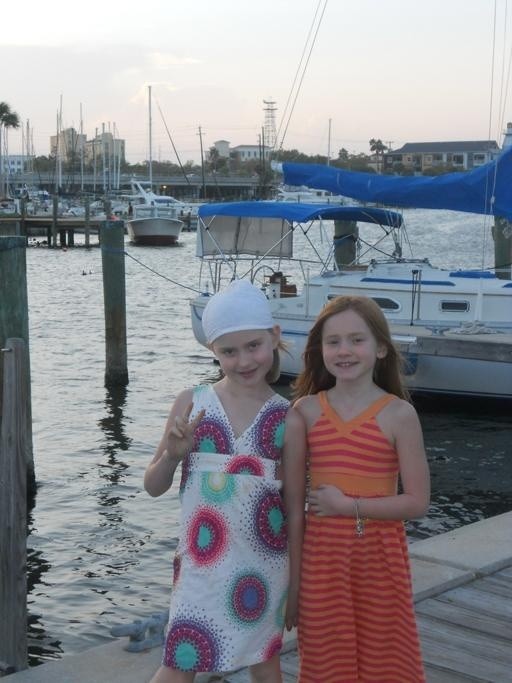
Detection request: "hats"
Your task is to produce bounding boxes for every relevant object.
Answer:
[201,279,276,349]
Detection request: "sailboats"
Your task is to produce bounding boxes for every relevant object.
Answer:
[270,120,359,205]
[0,83,207,244]
[179,0,512,417]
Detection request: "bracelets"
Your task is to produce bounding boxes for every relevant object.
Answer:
[352,497,366,536]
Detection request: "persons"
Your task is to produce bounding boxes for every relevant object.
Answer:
[290,294,431,683]
[128,202,134,216]
[144,276,307,683]
